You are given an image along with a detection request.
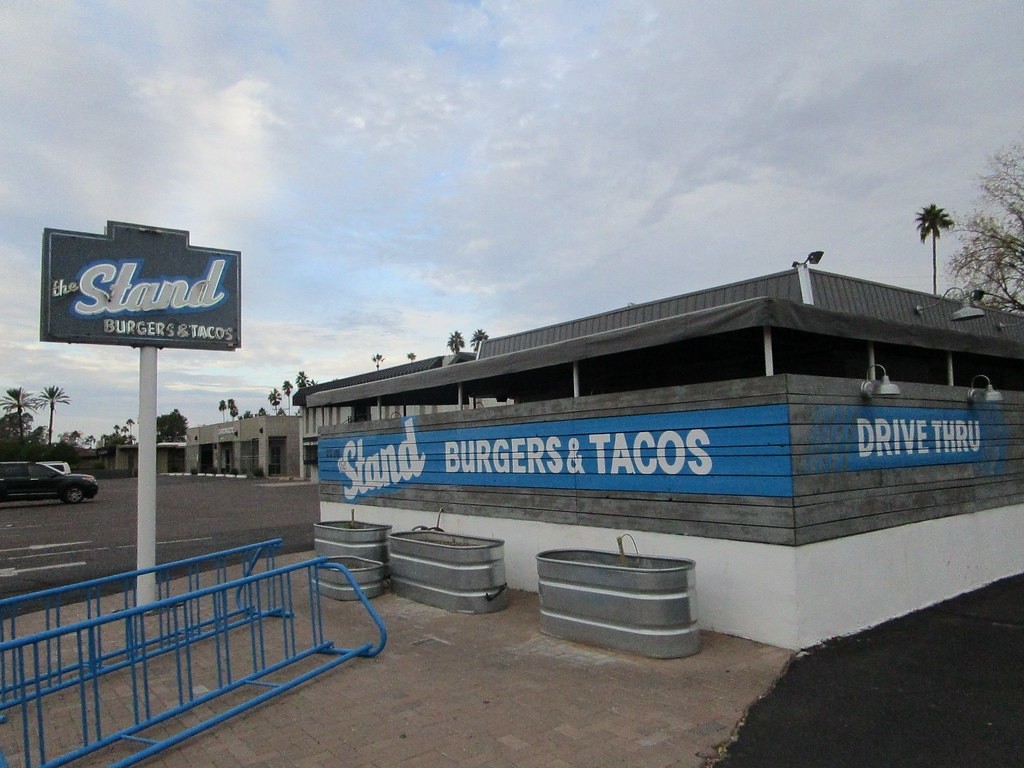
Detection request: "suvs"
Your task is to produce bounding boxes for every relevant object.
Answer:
[0,460,99,505]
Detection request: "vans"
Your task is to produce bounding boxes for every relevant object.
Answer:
[36,461,71,475]
